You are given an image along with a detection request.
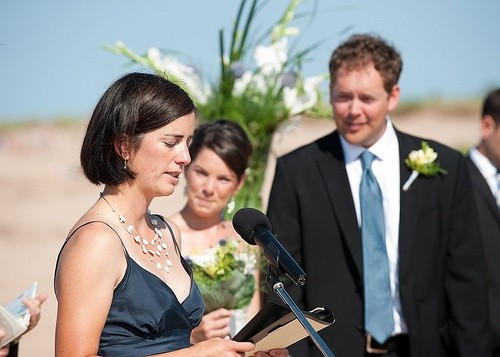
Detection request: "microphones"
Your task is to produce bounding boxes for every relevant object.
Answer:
[232,208,307,287]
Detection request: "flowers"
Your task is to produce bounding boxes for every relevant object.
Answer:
[105,0,353,230]
[186,241,259,314]
[404,142,447,191]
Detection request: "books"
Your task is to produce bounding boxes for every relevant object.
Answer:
[229,302,335,356]
[0,280,41,350]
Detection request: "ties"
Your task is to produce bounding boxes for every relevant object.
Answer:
[358,151,395,345]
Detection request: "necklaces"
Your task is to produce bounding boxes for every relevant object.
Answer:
[98,191,174,274]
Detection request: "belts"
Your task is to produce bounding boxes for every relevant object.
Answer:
[362,331,411,354]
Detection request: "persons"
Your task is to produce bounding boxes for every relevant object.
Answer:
[464,89,500,357]
[53,70,292,357]
[263,34,486,356]
[0,294,49,357]
[168,120,262,339]
